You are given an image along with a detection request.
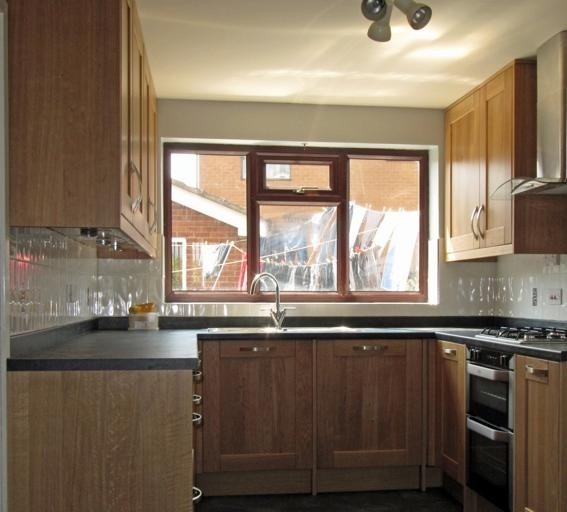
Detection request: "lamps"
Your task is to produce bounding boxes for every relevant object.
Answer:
[368,0,394,42]
[361,0,386,20]
[389,0,432,30]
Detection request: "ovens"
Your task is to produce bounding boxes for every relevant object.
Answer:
[464,345,517,512]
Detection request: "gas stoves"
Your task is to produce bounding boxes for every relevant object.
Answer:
[475,326,567,344]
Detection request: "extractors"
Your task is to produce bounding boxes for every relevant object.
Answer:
[488,29,567,200]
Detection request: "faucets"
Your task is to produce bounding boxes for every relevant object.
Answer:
[250,272,297,327]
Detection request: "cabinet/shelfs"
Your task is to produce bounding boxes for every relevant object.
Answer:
[434,339,468,487]
[202,342,313,473]
[512,354,567,512]
[443,57,537,264]
[316,339,424,470]
[7,349,204,512]
[6,1,158,260]
